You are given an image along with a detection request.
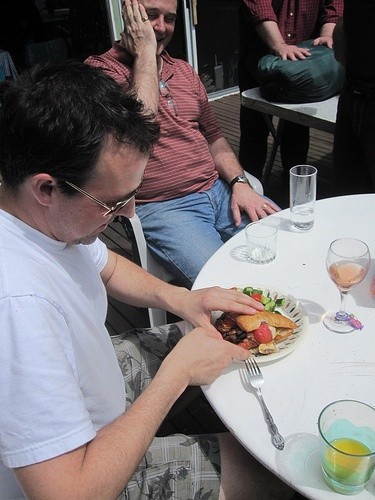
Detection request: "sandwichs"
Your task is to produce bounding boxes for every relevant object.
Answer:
[233,308,298,355]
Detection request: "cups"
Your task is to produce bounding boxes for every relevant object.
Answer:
[317,399,375,496]
[287,165,318,232]
[244,220,279,264]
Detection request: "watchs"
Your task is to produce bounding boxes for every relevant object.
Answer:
[230,175,248,188]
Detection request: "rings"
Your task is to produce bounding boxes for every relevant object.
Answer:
[262,204,268,208]
[142,17,149,22]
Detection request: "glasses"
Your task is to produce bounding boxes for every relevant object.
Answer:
[51,176,142,217]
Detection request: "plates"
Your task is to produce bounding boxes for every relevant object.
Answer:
[191,283,310,364]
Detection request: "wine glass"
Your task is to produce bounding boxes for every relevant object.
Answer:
[322,237,372,334]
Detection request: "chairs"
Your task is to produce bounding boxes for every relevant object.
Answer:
[121,171,264,328]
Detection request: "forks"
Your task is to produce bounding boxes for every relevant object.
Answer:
[244,358,284,451]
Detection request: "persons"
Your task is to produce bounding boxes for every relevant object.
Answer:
[240,1,343,179]
[84,0,282,290]
[0,60,295,500]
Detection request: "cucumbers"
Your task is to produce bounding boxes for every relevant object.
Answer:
[242,286,283,312]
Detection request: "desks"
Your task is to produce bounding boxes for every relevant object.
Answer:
[241,87,339,187]
[183,194,375,500]
